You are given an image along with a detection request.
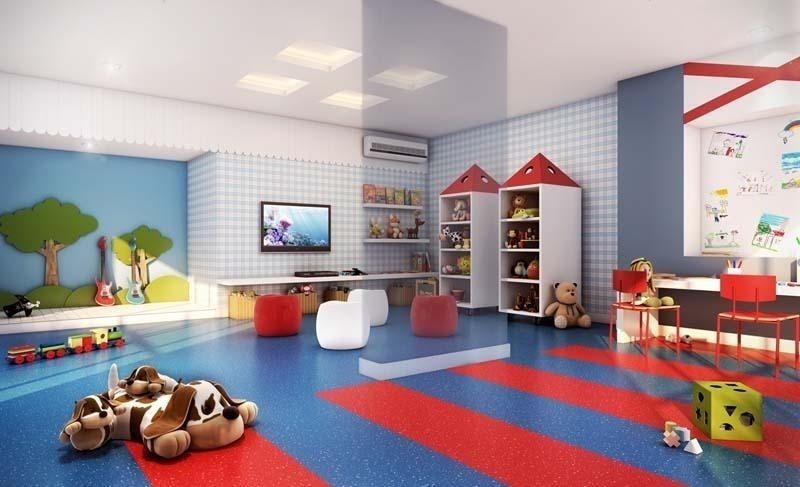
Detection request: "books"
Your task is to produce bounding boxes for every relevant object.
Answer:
[363,183,420,205]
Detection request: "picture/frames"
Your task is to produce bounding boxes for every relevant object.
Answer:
[415,280,436,296]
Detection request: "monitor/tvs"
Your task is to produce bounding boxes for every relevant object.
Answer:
[261,202,331,252]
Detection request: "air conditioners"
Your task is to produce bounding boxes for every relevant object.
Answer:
[363,135,429,164]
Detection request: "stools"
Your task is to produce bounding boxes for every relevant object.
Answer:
[410,293,459,337]
[315,299,372,350]
[347,287,389,327]
[253,293,302,338]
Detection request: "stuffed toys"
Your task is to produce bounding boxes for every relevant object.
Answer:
[61,364,259,460]
[438,195,539,312]
[630,258,675,307]
[545,282,592,330]
[289,283,313,296]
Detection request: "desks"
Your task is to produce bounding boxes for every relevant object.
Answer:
[615,271,800,344]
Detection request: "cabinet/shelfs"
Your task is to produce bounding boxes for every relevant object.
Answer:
[498,153,583,325]
[438,165,502,316]
[362,201,432,246]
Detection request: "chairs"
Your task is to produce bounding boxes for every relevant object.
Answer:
[609,270,681,359]
[714,273,800,379]
